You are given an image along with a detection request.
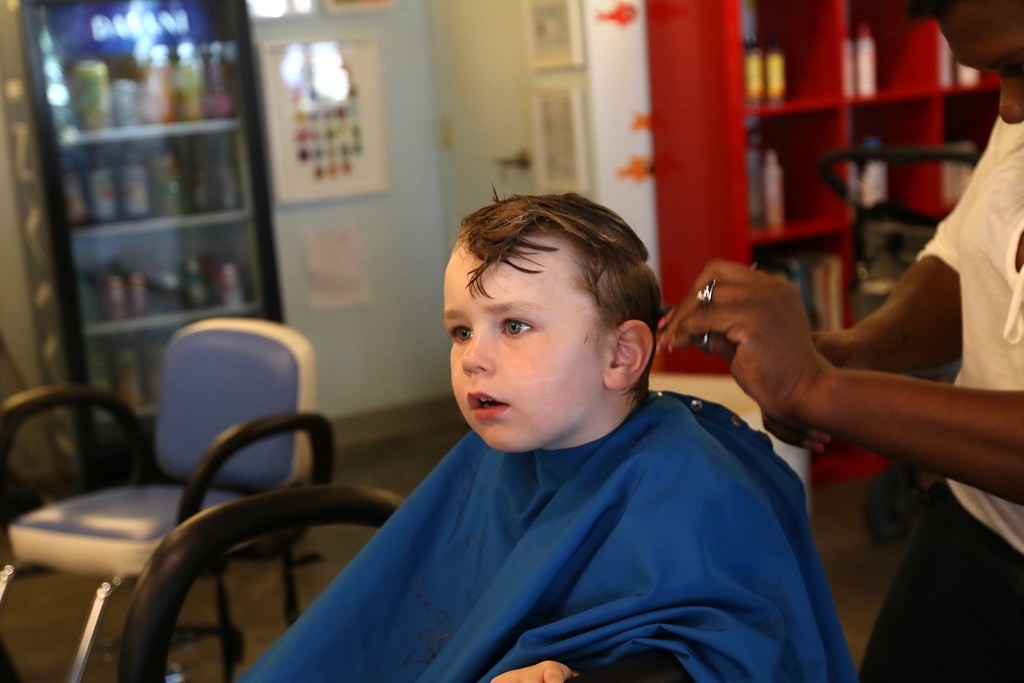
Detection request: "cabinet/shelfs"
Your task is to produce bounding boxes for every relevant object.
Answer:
[646,0,1003,486]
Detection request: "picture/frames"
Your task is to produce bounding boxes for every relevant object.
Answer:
[258,37,392,210]
[524,0,584,70]
[525,86,587,199]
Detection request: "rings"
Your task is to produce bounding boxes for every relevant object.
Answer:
[700,277,716,306]
[698,331,710,353]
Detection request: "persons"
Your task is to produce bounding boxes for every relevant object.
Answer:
[646,2,1024,683]
[234,187,865,679]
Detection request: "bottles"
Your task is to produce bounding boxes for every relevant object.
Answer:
[83,335,161,407]
[861,135,887,207]
[67,137,242,222]
[765,37,788,103]
[71,41,246,129]
[77,254,250,321]
[762,150,787,228]
[747,36,764,103]
[856,20,878,95]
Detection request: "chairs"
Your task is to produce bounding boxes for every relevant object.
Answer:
[0,316,312,683]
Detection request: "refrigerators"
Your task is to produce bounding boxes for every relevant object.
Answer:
[2,1,284,492]
